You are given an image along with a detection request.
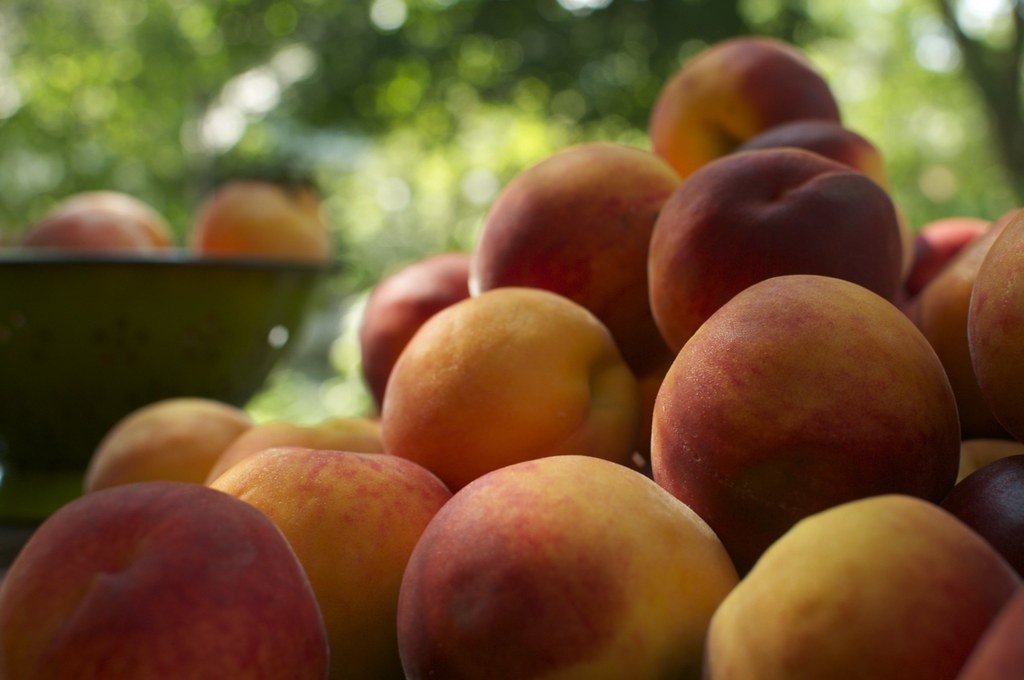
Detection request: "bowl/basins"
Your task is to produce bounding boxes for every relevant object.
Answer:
[0,247,341,528]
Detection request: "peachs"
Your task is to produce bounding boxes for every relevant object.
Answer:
[0,34,1024,680]
